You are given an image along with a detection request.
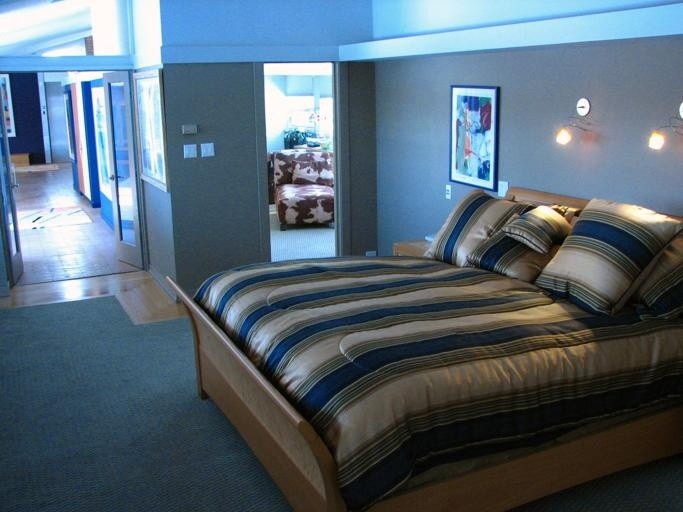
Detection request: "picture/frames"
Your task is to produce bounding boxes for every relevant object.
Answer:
[0,74,16,138]
[449,84,500,192]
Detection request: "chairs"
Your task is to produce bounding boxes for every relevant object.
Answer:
[267,149,335,231]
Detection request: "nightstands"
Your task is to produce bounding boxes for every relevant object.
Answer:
[392,241,431,257]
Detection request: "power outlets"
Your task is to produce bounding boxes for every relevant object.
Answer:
[446,185,451,199]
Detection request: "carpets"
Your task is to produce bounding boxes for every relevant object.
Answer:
[0,294,681,512]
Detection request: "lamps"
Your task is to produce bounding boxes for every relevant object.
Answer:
[649,102,683,151]
[555,97,590,145]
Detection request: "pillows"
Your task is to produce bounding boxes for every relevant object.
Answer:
[423,187,683,322]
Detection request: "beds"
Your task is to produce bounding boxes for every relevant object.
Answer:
[165,187,683,512]
